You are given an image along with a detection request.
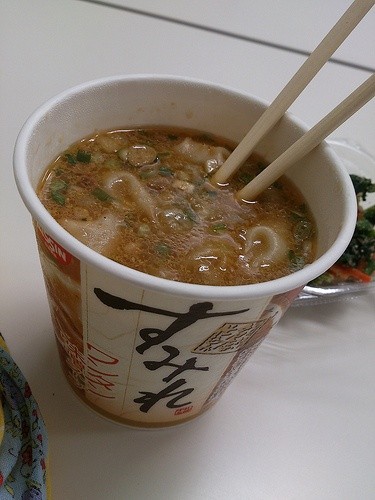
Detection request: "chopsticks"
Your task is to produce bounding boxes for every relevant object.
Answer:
[208,2,375,207]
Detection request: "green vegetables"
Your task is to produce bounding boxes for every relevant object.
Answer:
[334,174,375,275]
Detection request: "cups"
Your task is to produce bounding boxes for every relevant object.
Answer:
[13,74,360,431]
